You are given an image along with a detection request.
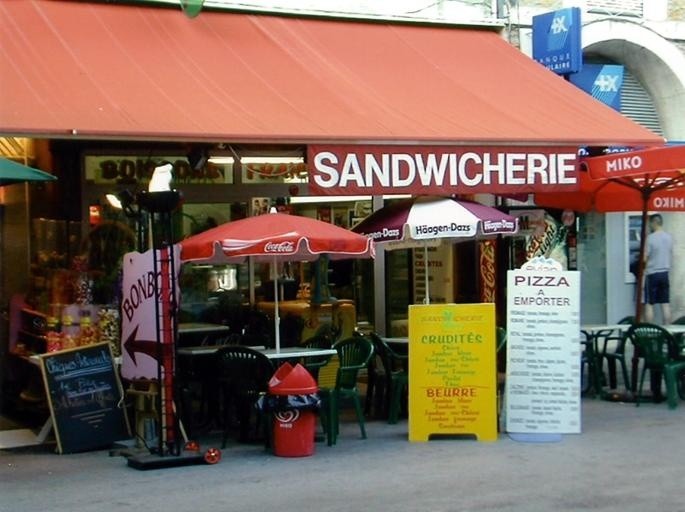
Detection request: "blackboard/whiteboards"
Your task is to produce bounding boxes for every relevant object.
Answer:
[39,341,133,454]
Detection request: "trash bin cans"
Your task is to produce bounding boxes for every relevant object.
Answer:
[269,362,319,457]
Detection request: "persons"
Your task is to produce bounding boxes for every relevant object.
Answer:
[641,213,674,328]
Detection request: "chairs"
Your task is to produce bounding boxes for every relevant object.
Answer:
[496,326,507,356]
[305,336,375,446]
[208,345,278,450]
[579,315,685,409]
[369,331,407,426]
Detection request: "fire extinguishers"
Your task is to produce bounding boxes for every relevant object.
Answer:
[567,231,578,269]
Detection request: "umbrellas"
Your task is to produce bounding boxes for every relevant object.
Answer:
[350,195,518,304]
[175,205,377,353]
[0,156,58,185]
[494,143,685,326]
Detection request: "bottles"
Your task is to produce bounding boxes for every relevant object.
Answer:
[45,316,95,353]
